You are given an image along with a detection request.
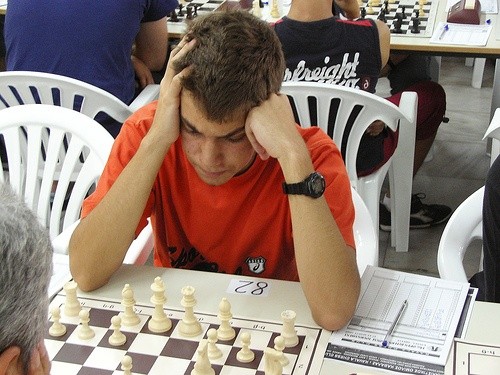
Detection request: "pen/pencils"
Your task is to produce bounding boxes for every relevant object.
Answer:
[382,299,407,346]
[439,24,448,39]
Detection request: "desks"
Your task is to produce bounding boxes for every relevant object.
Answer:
[167,0,500,157]
[43,255,500,375]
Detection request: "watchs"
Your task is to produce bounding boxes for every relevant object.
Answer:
[283,172,326,199]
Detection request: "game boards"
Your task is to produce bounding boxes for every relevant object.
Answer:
[43,293,338,375]
[339,0,439,38]
[165,0,227,35]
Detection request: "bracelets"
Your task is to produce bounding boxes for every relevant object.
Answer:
[387,57,395,67]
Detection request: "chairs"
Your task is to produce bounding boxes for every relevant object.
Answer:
[436,185,486,284]
[277,81,418,252]
[0,71,160,255]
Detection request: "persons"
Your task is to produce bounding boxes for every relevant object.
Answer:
[272,0,453,231]
[469,154,500,303]
[0,0,179,221]
[0,180,54,375]
[69,9,360,331]
[378,52,432,95]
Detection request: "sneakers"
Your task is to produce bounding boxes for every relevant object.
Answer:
[378,191,453,232]
